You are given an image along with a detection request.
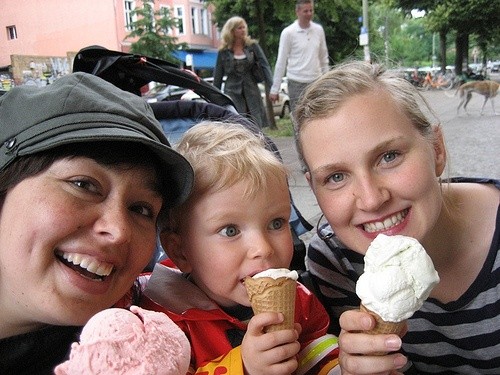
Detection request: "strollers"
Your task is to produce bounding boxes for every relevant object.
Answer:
[70,44,317,280]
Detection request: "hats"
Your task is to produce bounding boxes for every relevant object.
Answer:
[0,72,192,211]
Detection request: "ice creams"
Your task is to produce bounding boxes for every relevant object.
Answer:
[51,305,192,375]
[243,268,299,334]
[355,233,441,355]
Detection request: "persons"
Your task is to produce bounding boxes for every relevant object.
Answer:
[115,120,342,375]
[289,59,500,375]
[269,0,331,173]
[211,16,273,130]
[0,72,195,375]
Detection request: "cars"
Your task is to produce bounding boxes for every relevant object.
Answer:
[182,81,289,120]
[143,77,216,104]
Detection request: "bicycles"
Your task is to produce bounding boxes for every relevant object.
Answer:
[422,68,462,91]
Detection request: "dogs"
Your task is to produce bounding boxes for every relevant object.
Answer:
[456,80,500,117]
